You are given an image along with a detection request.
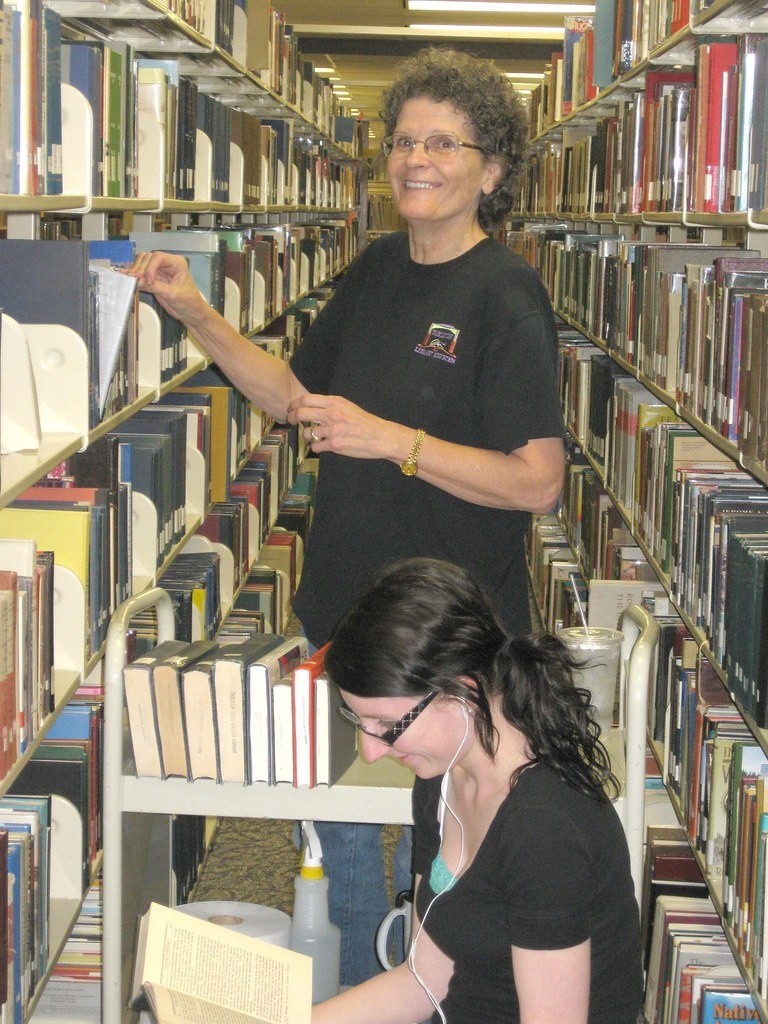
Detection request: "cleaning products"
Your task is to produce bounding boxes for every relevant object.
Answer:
[288,822,341,1006]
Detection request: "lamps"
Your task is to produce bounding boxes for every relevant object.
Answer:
[404,0,592,17]
[405,23,565,34]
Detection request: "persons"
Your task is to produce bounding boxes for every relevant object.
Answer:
[116,48,566,982]
[312,558,646,1024]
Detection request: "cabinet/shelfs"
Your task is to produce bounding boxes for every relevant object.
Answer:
[0,0,768,1024]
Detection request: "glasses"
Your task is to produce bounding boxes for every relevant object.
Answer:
[382,135,487,161]
[337,686,442,743]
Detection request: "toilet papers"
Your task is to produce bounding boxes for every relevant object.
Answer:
[171,900,292,946]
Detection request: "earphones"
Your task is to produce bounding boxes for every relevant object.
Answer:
[456,695,468,719]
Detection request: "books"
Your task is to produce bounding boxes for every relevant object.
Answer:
[0,0,768,1024]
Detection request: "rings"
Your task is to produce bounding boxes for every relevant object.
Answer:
[310,425,322,440]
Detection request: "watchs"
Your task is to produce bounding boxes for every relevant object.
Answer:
[400,429,427,476]
